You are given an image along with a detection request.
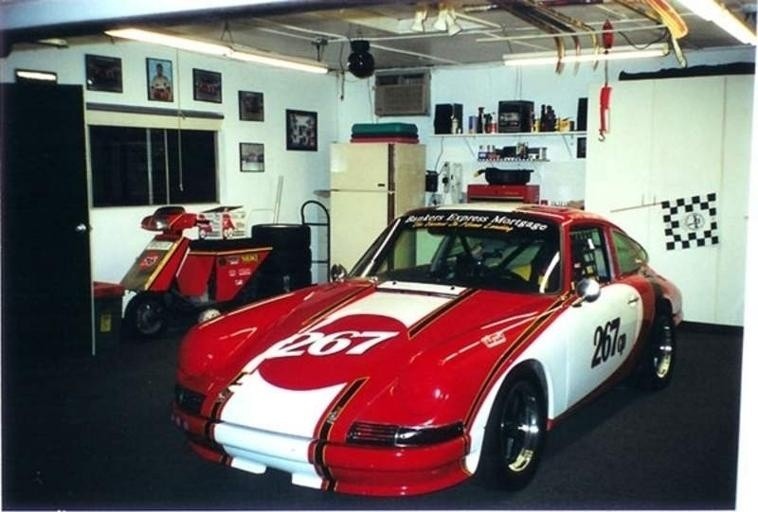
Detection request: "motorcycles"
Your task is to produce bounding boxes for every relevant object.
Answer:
[120,206,302,341]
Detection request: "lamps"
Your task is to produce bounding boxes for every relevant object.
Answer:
[500,43,671,67]
[410,7,462,37]
[105,25,329,75]
[345,41,375,78]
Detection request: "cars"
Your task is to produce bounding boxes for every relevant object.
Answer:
[169,202,682,498]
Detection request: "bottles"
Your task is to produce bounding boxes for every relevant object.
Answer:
[477,106,497,133]
[539,104,555,131]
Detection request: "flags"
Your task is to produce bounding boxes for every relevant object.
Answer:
[658,192,719,250]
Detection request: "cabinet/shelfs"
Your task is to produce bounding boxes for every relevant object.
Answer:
[427,130,587,163]
[584,73,757,334]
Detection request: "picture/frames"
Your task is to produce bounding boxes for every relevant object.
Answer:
[192,67,222,104]
[240,141,265,173]
[85,53,123,93]
[286,108,318,153]
[145,56,174,104]
[238,89,265,122]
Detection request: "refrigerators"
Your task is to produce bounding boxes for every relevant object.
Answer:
[329,142,426,277]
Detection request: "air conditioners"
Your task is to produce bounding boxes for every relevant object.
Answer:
[373,68,431,115]
